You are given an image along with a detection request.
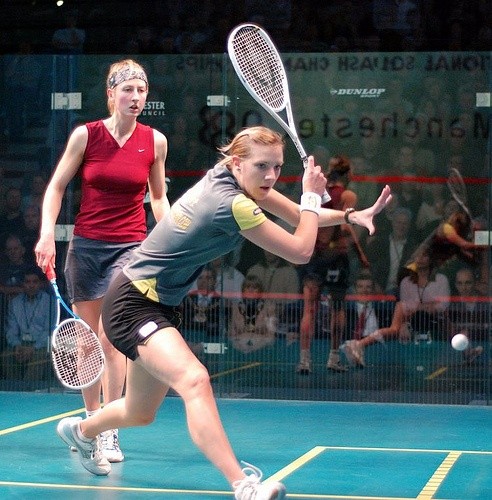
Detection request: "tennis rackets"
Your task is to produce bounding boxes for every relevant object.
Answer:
[226,23,334,207]
[42,260,105,389]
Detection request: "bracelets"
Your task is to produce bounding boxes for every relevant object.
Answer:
[344,208,355,225]
[299,192,321,216]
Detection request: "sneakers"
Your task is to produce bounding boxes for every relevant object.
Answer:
[297,358,310,374]
[339,340,365,369]
[328,356,349,372]
[97,428,123,462]
[233,461,287,500]
[463,346,481,365]
[58,416,111,475]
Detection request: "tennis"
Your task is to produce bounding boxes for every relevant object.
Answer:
[450,333,468,351]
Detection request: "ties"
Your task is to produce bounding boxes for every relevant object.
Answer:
[352,306,367,340]
[199,298,206,314]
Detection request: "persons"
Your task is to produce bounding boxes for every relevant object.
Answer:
[0,0,492,394]
[296,150,369,373]
[56,127,393,500]
[34,59,170,462]
[341,207,489,369]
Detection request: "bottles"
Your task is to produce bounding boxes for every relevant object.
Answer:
[22,331,32,347]
